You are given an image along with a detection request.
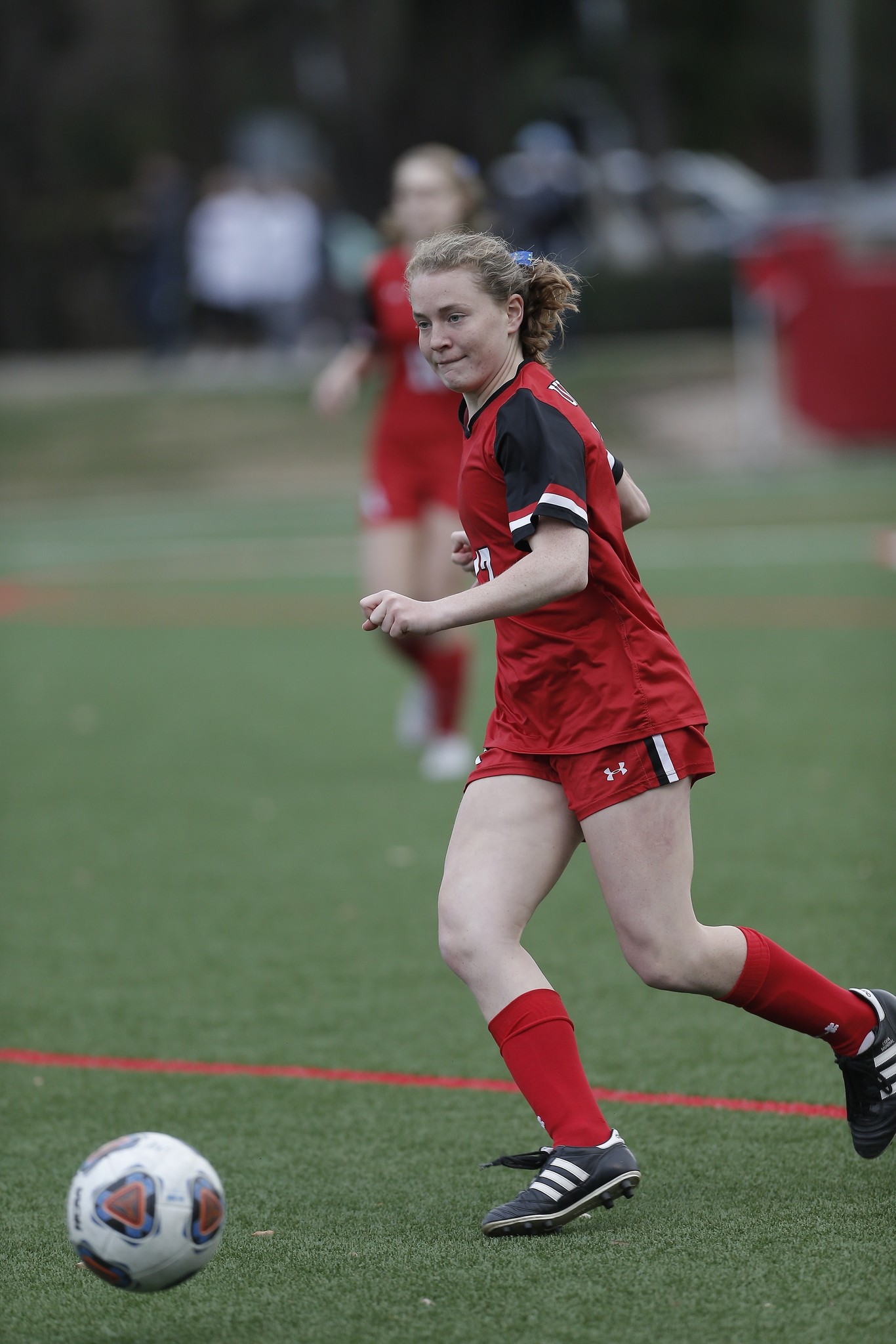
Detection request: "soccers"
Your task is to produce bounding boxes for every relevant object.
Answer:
[67,1133,226,1294]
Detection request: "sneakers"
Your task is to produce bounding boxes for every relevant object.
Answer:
[834,988,895,1160]
[480,1128,641,1237]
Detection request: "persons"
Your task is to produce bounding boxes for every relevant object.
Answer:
[314,142,491,777]
[145,101,598,386]
[357,231,896,1236]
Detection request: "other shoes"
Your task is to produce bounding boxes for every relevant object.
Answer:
[394,684,475,783]
